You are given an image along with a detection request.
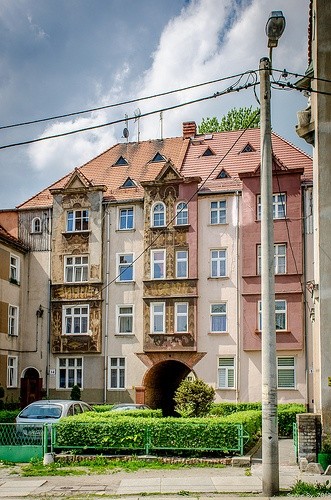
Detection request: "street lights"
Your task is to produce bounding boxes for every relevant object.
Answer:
[261,9,286,498]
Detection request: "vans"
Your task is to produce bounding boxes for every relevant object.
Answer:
[16,400,97,444]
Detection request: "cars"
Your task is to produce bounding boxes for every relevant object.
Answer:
[111,404,151,410]
